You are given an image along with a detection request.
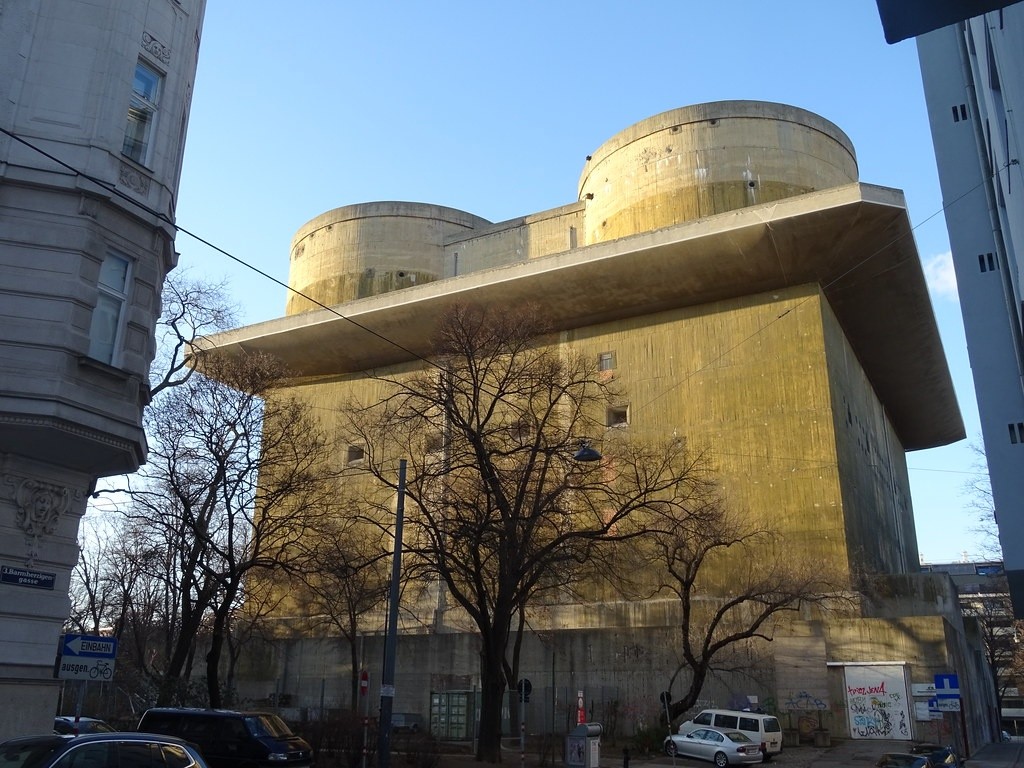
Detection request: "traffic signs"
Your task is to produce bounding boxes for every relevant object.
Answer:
[58,633,118,682]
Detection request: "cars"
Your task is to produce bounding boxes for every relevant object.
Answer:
[664,727,763,768]
[0,716,209,768]
[874,743,969,768]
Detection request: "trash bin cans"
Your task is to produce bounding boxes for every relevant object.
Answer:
[565,723,603,768]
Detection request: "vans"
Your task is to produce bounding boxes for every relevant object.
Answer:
[136,707,314,768]
[678,708,785,762]
